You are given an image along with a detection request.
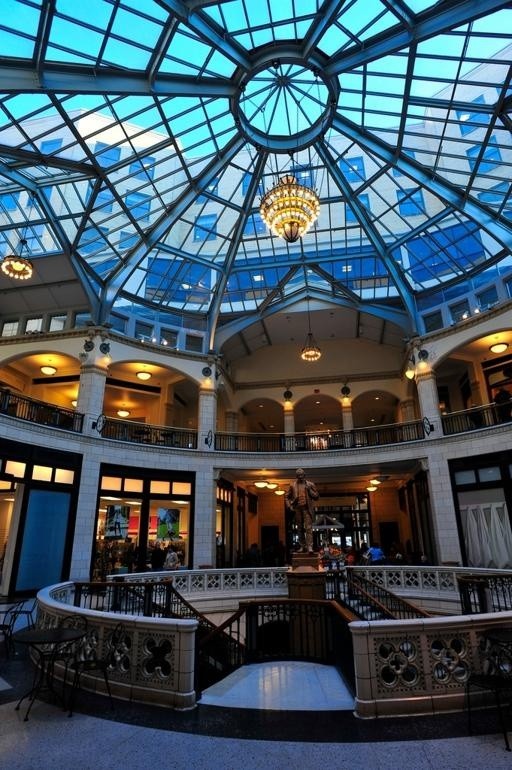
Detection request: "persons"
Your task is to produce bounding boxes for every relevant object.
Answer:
[113,509,122,536]
[471,403,483,429]
[95,535,185,573]
[216,532,223,560]
[494,386,512,423]
[285,468,319,553]
[162,520,174,541]
[237,540,427,567]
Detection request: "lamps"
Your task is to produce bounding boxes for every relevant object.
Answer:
[40,366,57,375]
[418,348,428,370]
[136,372,152,381]
[202,367,211,387]
[406,357,415,380]
[259,175,320,243]
[301,298,322,361]
[79,338,92,362]
[100,342,112,364]
[283,392,292,410]
[1,198,33,280]
[116,410,130,418]
[217,372,226,389]
[72,400,78,407]
[489,342,509,353]
[342,386,350,403]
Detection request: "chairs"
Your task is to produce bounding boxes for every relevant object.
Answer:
[465,627,512,753]
[0,599,124,722]
